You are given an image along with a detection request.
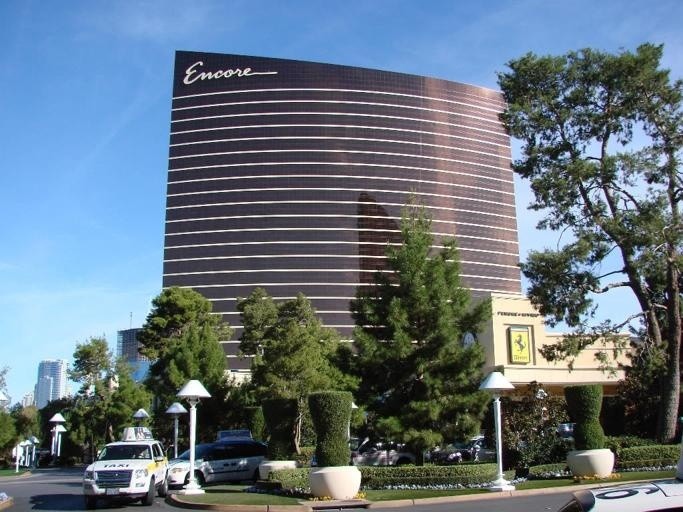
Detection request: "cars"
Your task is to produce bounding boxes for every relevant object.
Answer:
[558,479,682,512]
[346,437,418,466]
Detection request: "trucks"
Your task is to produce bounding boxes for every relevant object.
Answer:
[216,429,253,441]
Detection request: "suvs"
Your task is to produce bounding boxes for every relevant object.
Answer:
[167,441,267,489]
[83,440,170,509]
[430,436,485,464]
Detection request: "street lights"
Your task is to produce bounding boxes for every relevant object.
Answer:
[165,402,188,458]
[176,380,212,495]
[132,408,150,427]
[16,435,39,472]
[478,371,515,486]
[49,413,67,458]
